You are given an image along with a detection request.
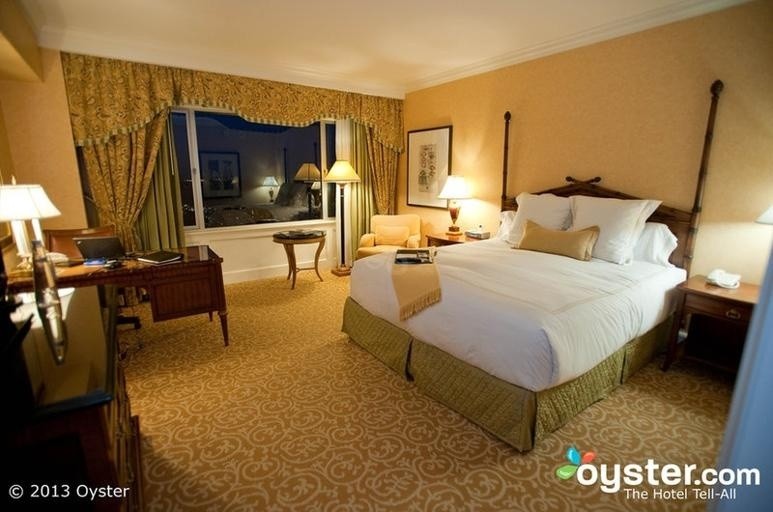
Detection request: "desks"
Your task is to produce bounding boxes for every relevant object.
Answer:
[0,245,232,348]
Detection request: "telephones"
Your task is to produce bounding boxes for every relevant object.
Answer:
[706,269,742,291]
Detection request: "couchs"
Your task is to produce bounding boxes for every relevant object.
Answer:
[358,214,421,260]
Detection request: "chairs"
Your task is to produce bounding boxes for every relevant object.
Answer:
[41,225,141,329]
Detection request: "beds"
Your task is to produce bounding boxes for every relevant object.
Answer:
[184,141,320,230]
[339,79,725,456]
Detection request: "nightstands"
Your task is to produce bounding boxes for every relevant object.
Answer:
[425,231,484,247]
[658,275,762,381]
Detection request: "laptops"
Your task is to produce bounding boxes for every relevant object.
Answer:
[72,236,136,260]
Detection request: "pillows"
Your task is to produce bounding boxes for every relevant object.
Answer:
[511,220,601,261]
[374,225,410,246]
[566,195,663,265]
[503,193,573,248]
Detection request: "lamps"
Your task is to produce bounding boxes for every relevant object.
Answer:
[324,160,359,277]
[438,175,474,236]
[263,176,278,202]
[755,201,773,238]
[0,183,62,272]
[294,162,321,217]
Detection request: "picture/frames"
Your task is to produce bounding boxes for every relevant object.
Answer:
[200,151,242,200]
[407,125,453,211]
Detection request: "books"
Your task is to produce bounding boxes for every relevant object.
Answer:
[395,249,433,264]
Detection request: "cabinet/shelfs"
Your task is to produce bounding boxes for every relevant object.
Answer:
[0,282,145,512]
